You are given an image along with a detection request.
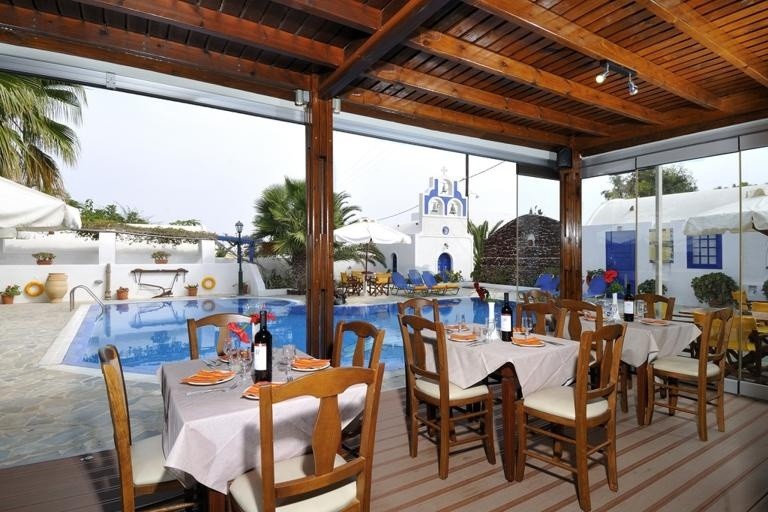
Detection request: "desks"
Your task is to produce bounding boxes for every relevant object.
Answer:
[552,310,703,421]
[158,348,370,510]
[418,324,588,451]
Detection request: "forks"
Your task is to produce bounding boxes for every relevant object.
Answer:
[198,356,219,367]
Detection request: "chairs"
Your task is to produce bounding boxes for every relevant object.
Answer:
[183,314,261,358]
[583,275,607,305]
[518,320,626,512]
[518,274,554,300]
[97,346,227,509]
[396,297,704,360]
[642,306,738,441]
[398,309,498,480]
[340,270,460,297]
[326,321,383,452]
[229,361,387,511]
[549,278,561,296]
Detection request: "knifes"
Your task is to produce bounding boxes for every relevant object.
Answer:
[545,339,563,346]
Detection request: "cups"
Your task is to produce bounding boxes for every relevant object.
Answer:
[473,324,482,337]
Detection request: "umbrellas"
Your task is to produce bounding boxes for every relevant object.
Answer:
[0,176,82,230]
[683,193,768,237]
[334,221,413,273]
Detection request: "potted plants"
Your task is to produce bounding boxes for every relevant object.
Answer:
[691,273,739,307]
[0,285,22,304]
[184,283,199,296]
[637,280,667,296]
[32,252,56,265]
[152,251,171,264]
[608,283,624,299]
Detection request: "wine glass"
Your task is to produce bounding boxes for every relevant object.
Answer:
[521,315,534,337]
[456,313,466,333]
[484,317,497,342]
[223,330,296,383]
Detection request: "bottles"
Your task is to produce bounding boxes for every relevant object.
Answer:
[500,292,513,343]
[624,283,634,321]
[252,309,273,383]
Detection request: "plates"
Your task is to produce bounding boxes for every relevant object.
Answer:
[445,323,469,332]
[511,336,543,348]
[583,315,606,322]
[242,381,281,399]
[219,351,231,363]
[291,358,330,371]
[640,318,669,326]
[446,333,478,341]
[188,369,236,385]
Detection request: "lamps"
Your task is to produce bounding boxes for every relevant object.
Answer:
[595,60,638,95]
[294,90,309,106]
[333,98,341,113]
